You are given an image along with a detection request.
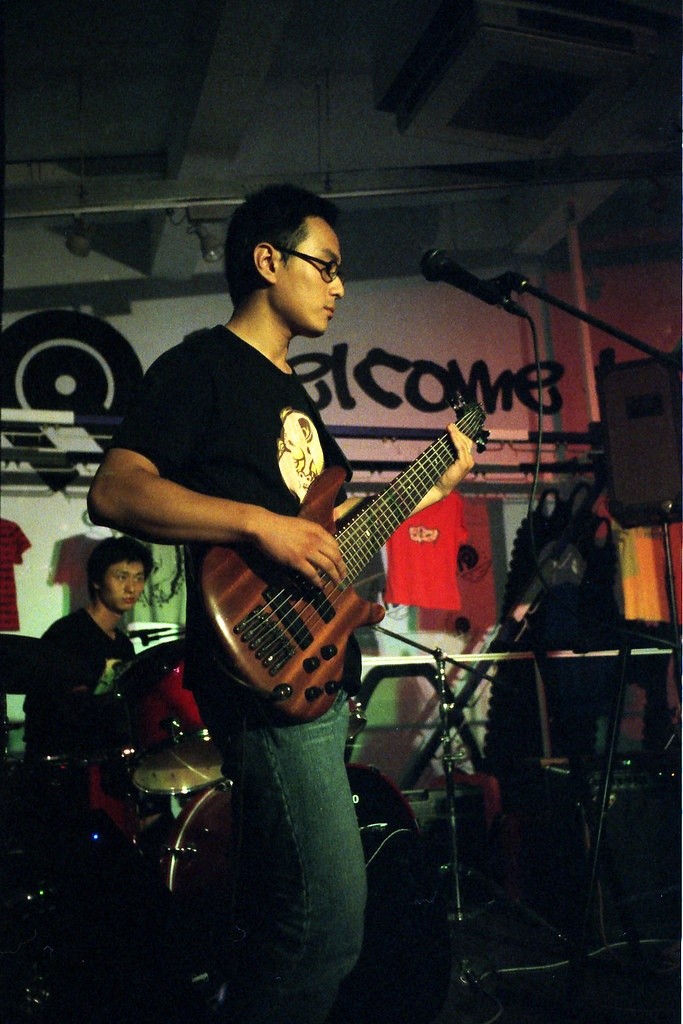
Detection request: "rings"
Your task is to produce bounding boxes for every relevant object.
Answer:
[468,448,471,454]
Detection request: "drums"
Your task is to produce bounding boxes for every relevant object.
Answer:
[162,760,235,897]
[112,638,229,798]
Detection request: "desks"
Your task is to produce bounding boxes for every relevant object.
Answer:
[360,650,677,985]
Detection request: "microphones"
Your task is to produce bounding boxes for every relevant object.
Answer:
[124,628,168,639]
[419,248,529,319]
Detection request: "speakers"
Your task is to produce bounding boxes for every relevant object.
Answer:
[569,769,681,952]
[595,356,683,530]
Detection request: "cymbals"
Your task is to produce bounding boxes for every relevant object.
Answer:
[2,633,53,693]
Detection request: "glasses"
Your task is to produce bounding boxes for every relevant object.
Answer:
[249,239,346,285]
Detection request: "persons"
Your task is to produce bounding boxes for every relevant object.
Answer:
[19,532,154,819]
[85,182,478,1024]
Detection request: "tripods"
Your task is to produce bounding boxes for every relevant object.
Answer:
[373,628,575,948]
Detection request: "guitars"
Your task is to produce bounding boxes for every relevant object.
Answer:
[191,391,491,723]
